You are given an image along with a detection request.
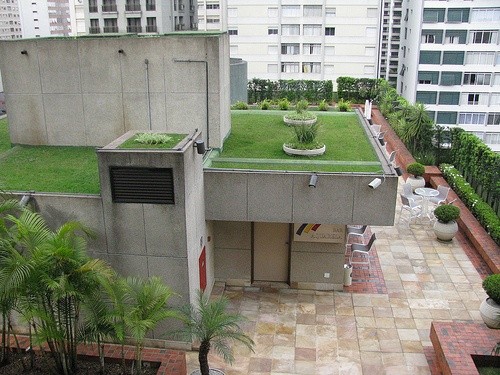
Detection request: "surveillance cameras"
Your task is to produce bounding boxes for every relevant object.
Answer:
[368,178,381,189]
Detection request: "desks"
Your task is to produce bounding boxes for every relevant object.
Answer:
[415,188,440,217]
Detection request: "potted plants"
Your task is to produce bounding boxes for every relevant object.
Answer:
[434,204,459,240]
[480,273,500,329]
[406,162,426,193]
[335,97,351,112]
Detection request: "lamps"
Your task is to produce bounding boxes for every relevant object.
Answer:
[118,49,123,53]
[395,165,402,177]
[20,50,27,55]
[308,174,318,189]
[379,138,385,145]
[192,140,204,154]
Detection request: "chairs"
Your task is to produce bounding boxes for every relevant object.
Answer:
[398,183,458,226]
[371,124,385,140]
[347,225,368,245]
[348,233,375,268]
[389,150,397,167]
